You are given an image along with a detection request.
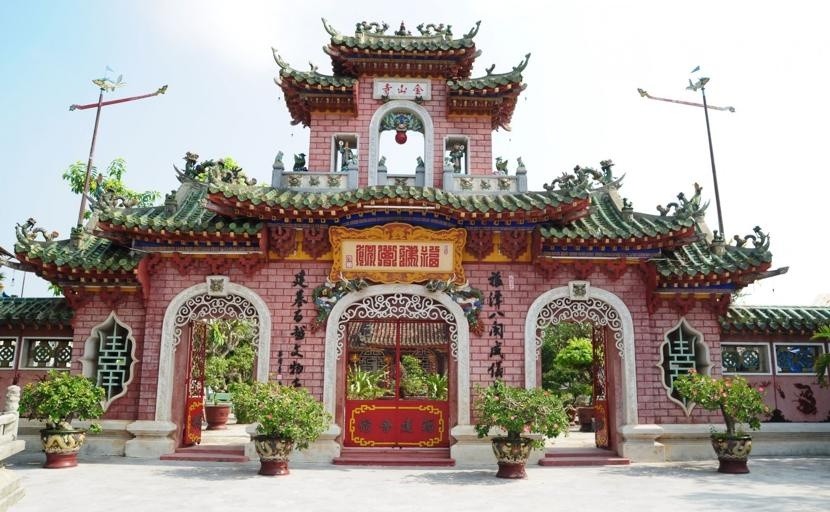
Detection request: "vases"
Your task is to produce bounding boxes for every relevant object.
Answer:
[490,436,534,480]
[711,431,754,474]
[250,434,295,475]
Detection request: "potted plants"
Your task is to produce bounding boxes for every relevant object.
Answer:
[553,335,599,431]
[345,354,448,403]
[18,368,108,468]
[198,319,247,428]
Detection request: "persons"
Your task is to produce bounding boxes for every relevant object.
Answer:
[449,143,465,173]
[339,140,353,172]
[381,350,408,397]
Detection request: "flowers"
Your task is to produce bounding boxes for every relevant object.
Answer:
[229,379,333,451]
[473,380,570,451]
[674,366,775,437]
